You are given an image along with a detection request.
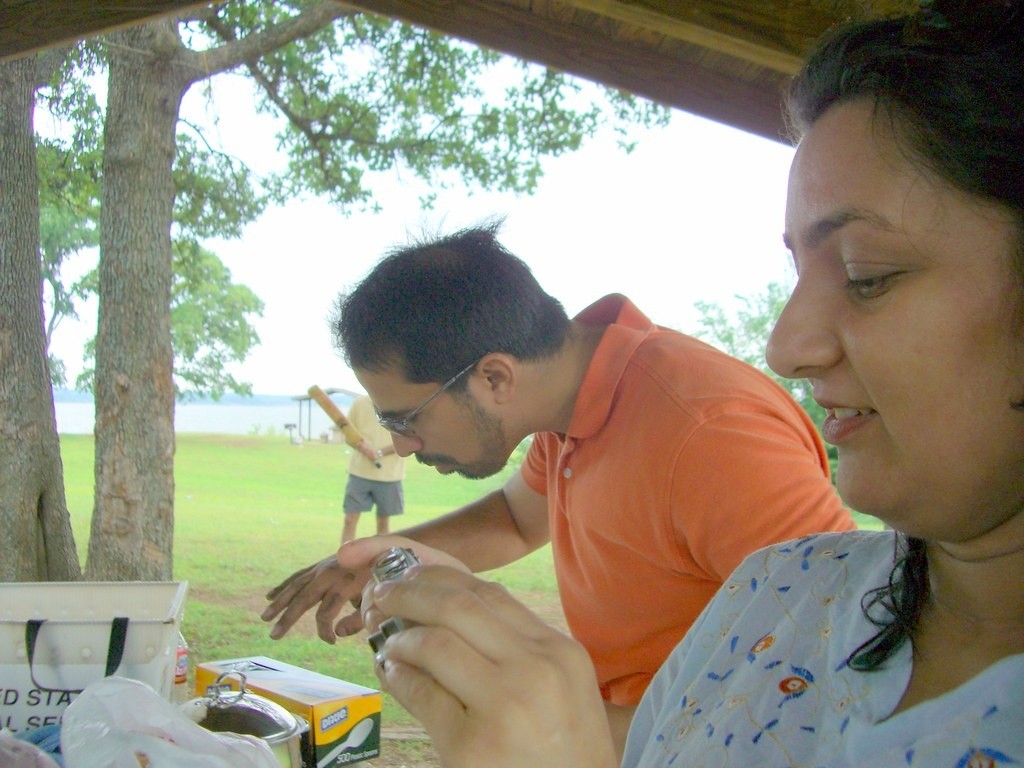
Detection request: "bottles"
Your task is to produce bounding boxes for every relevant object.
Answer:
[172,631,188,706]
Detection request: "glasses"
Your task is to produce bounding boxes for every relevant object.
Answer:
[373,360,479,438]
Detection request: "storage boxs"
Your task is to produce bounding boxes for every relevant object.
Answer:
[0,579,189,735]
[193,655,383,768]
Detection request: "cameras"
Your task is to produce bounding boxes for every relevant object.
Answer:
[366,546,421,670]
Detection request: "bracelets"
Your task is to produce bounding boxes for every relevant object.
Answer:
[378,450,383,457]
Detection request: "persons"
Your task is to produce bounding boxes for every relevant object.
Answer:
[261,220,857,768]
[337,0,1024,768]
[341,395,404,545]
